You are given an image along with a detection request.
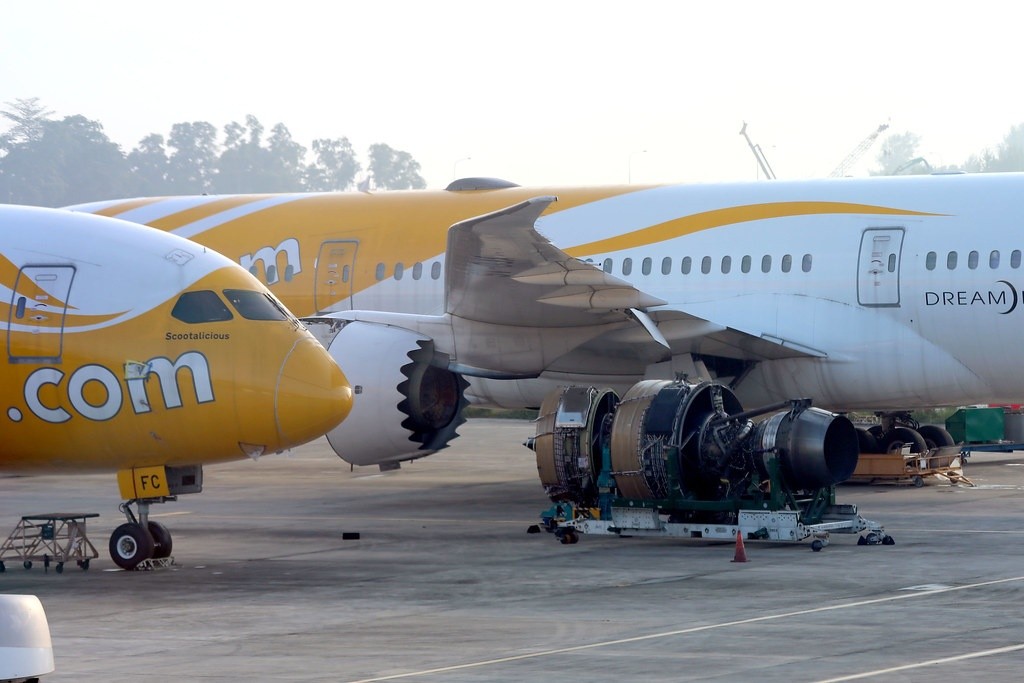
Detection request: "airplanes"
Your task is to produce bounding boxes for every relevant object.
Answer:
[0,202,355,568]
[44,168,1023,545]
